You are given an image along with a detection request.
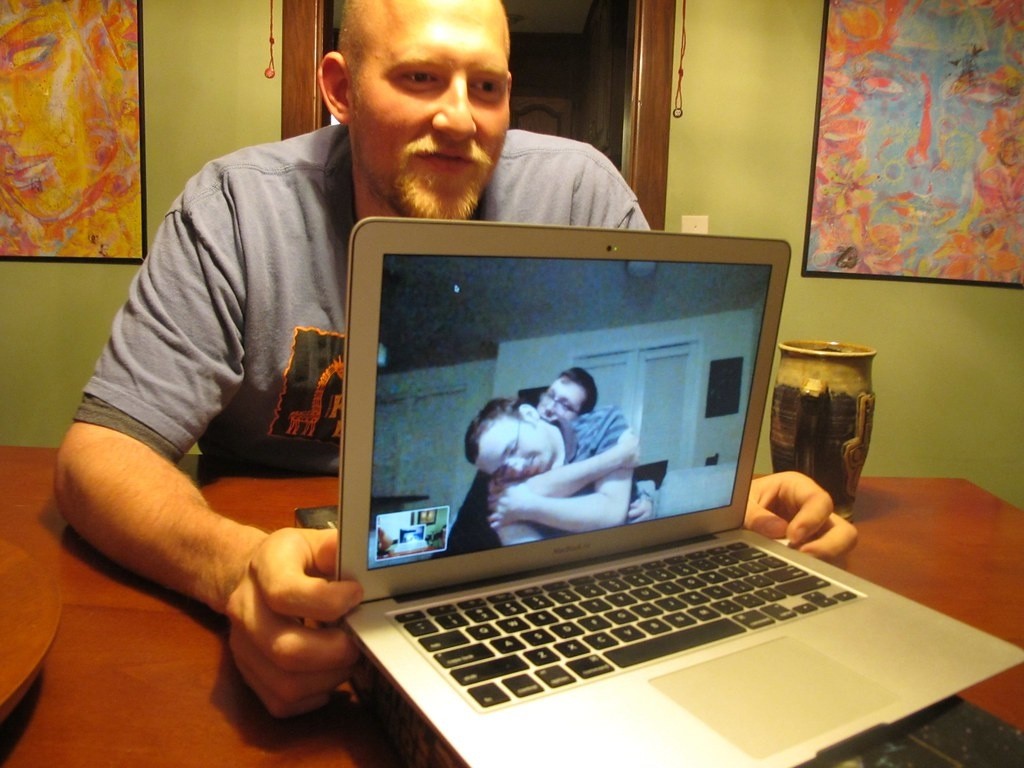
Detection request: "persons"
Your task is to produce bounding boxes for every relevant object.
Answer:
[53,0,860,718]
[441,366,656,555]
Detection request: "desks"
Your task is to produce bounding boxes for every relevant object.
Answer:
[0,446,1024,768]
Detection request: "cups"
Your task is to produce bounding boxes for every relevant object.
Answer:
[769,339,877,523]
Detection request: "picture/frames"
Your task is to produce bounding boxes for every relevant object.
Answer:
[800,1,1023,290]
[0,0,148,265]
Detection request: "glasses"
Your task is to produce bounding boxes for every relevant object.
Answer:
[492,422,520,480]
[542,389,580,414]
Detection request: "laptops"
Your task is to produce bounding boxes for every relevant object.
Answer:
[337,217,1024,768]
[394,524,428,553]
